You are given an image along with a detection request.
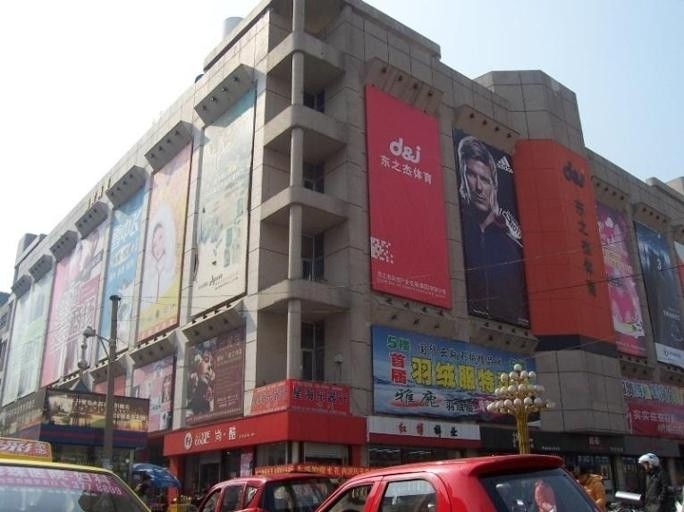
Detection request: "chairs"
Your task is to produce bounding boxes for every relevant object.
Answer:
[274,499,288,512]
[298,496,314,507]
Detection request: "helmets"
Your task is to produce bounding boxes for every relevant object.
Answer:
[638,453,659,469]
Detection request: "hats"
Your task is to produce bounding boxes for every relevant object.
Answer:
[575,465,590,479]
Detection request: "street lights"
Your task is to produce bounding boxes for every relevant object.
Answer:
[488,365,556,454]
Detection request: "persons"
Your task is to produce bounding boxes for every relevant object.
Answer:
[496,452,684,512]
[458,135,529,324]
[185,349,214,418]
[141,224,176,298]
[135,477,214,511]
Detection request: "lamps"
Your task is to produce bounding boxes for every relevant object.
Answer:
[333,353,343,363]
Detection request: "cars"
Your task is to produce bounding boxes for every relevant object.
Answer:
[0,437,151,512]
